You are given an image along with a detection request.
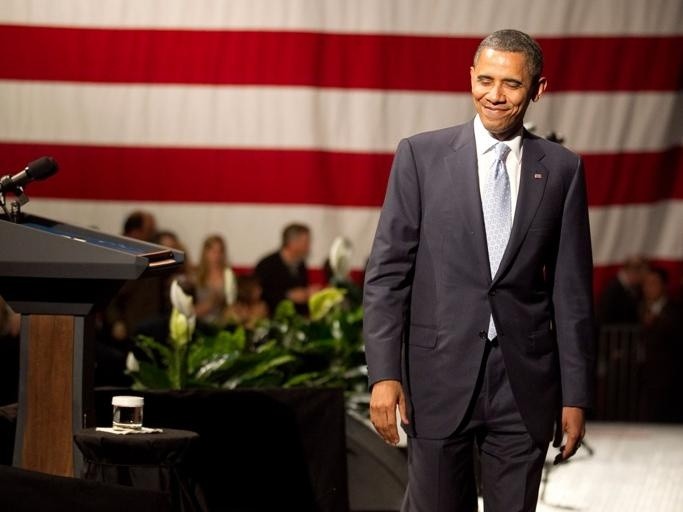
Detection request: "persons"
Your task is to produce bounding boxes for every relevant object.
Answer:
[85,210,364,368]
[598,256,653,326]
[644,267,679,333]
[364,31,595,512]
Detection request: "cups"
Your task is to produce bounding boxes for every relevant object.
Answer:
[112,396,144,431]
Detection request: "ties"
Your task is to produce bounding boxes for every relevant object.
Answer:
[485,141,512,341]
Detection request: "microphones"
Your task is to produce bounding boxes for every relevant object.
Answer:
[0,156,59,192]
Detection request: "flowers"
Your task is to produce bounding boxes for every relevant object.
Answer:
[124,236,371,420]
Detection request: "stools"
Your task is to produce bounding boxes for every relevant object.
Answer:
[74,427,209,512]
